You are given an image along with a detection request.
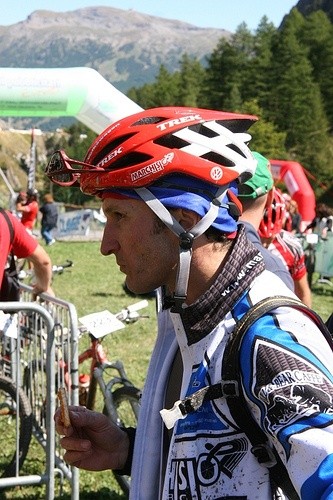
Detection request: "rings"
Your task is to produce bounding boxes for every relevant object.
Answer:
[82,406,86,412]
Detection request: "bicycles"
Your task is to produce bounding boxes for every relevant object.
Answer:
[0,308,33,492]
[23,298,150,498]
[19,260,75,359]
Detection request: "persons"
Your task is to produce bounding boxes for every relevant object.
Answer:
[54,110,333,500]
[40,193,58,246]
[236,151,333,310]
[0,207,55,410]
[16,188,39,236]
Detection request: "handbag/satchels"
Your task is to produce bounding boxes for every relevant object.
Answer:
[0,208,20,314]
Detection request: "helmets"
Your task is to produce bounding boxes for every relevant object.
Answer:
[27,189,38,196]
[80,106,261,196]
[257,191,286,239]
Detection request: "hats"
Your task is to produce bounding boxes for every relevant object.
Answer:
[237,151,274,199]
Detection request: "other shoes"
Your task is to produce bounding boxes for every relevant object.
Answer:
[48,238,56,246]
